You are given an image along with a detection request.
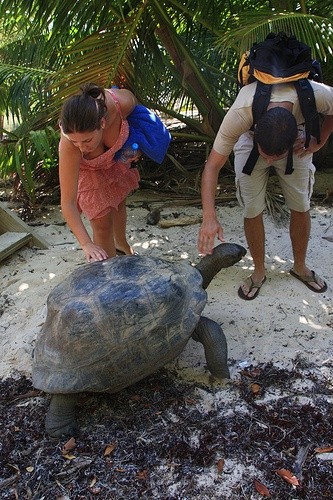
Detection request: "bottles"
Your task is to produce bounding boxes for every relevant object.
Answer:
[120,143,138,164]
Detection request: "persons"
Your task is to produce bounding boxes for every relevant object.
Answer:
[198,80,333,300]
[58,83,140,265]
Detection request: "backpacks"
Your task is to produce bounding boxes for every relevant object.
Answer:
[238,31,322,175]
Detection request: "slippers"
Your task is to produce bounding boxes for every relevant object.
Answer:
[238,274,266,301]
[115,245,139,257]
[290,266,328,293]
